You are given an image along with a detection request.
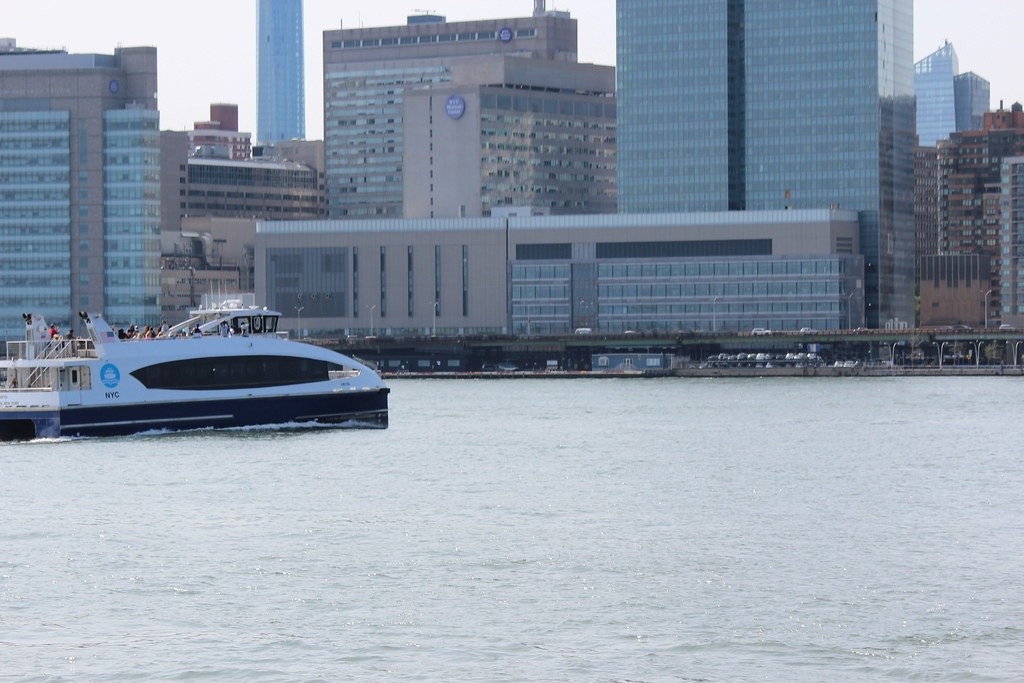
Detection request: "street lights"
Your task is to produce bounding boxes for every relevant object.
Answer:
[366,305,377,337]
[884,342,899,371]
[713,296,719,332]
[294,305,304,339]
[984,289,992,332]
[970,341,985,369]
[432,302,438,337]
[848,293,853,333]
[932,341,949,370]
[1006,341,1023,368]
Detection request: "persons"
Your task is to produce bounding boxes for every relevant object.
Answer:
[118,320,202,339]
[220,322,249,337]
[66,329,75,340]
[49,324,59,338]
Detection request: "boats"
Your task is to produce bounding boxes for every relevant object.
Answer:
[0,255,391,443]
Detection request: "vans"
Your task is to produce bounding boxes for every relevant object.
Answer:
[575,328,593,334]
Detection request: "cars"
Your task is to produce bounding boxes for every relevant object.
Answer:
[999,324,1015,331]
[800,327,818,336]
[853,326,875,335]
[706,352,858,370]
[952,324,972,334]
[624,330,635,334]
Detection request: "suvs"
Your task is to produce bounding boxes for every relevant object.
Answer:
[751,328,772,336]
